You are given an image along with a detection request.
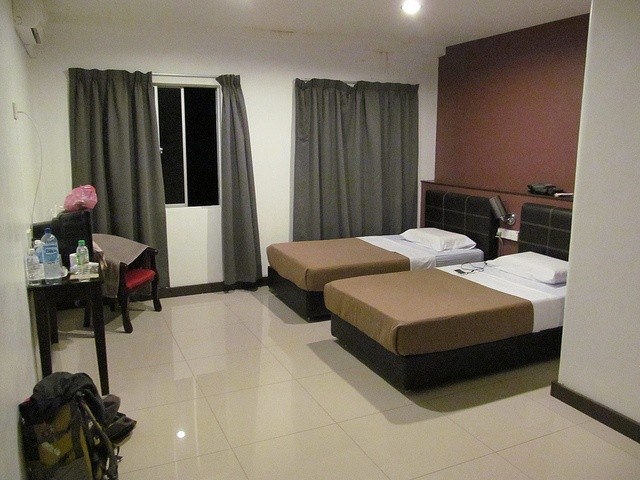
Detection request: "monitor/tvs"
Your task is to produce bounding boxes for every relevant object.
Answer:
[32,208,94,270]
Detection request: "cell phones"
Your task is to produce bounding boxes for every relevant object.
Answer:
[455,268,466,275]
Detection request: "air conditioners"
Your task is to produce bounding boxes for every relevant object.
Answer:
[12,0,46,58]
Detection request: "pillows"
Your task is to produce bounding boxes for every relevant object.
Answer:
[399,228,477,252]
[491,250,569,284]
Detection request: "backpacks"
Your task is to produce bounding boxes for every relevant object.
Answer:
[19,389,137,480]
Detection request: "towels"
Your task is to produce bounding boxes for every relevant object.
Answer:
[91,234,148,300]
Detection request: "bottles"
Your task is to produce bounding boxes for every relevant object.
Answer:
[39,228,63,285]
[34,240,43,262]
[24,248,40,286]
[76,240,90,283]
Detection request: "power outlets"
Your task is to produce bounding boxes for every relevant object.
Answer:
[12,103,20,121]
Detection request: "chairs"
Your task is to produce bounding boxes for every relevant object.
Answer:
[83,233,163,334]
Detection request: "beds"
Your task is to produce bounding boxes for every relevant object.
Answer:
[323,203,572,395]
[267,190,500,321]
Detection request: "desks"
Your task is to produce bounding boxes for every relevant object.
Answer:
[27,257,110,397]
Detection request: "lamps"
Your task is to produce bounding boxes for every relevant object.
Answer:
[489,196,516,226]
